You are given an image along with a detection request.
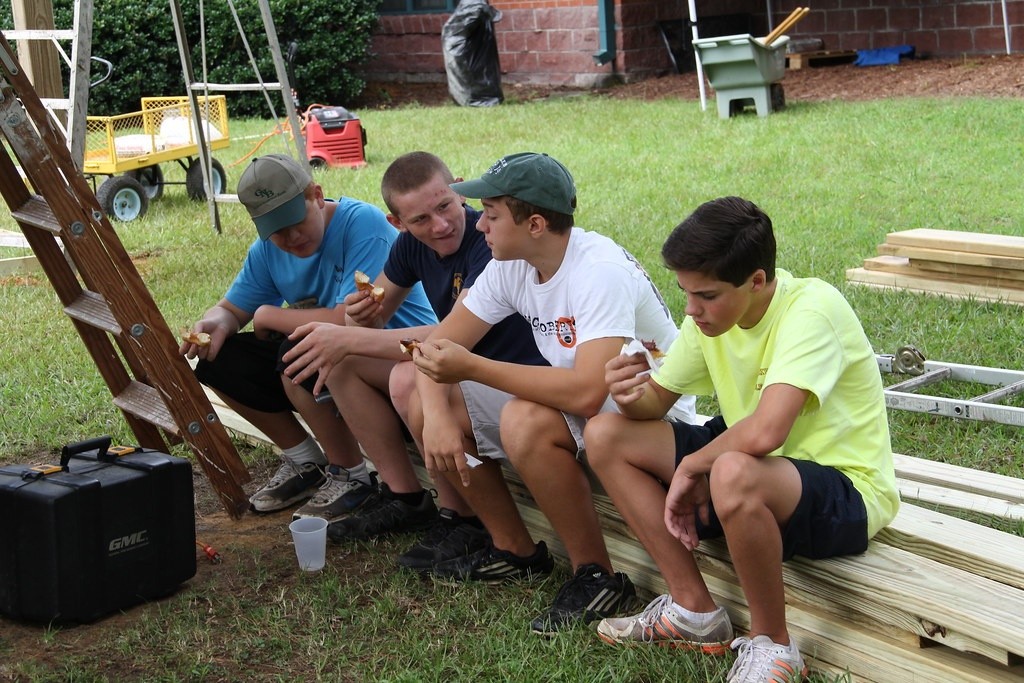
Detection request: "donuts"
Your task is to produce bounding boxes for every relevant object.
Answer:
[398,338,439,359]
[180,330,211,346]
[640,339,669,359]
[355,270,385,303]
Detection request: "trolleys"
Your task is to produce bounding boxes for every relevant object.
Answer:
[84,55,231,222]
[692,33,791,119]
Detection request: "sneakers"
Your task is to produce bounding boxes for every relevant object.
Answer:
[597,593,735,656]
[395,508,491,573]
[727,634,808,683]
[430,539,555,587]
[246,452,329,513]
[531,564,637,637]
[291,462,378,525]
[327,482,439,546]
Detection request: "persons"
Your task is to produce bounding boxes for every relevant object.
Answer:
[583,196,900,683]
[407,152,695,635]
[282,151,553,568]
[178,154,437,529]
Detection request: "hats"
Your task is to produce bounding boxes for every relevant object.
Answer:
[238,153,312,243]
[448,151,577,216]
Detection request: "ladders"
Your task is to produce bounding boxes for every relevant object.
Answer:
[874,346,1024,426]
[0,0,321,294]
[0,31,255,522]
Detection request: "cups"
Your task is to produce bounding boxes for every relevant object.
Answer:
[288,516,328,571]
